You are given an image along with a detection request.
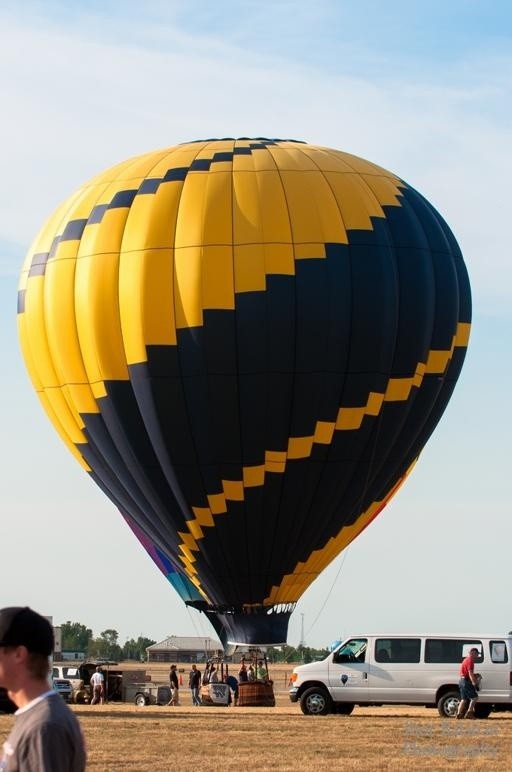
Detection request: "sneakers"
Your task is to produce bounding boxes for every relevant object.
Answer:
[456,711,478,719]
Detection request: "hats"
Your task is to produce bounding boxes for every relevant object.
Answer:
[0,605,55,656]
[469,648,482,654]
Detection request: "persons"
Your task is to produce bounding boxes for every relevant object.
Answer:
[1,605,87,772]
[253,661,269,681]
[88,666,106,705]
[454,647,483,720]
[188,664,202,705]
[238,665,248,681]
[162,664,180,707]
[207,666,218,682]
[246,665,257,683]
